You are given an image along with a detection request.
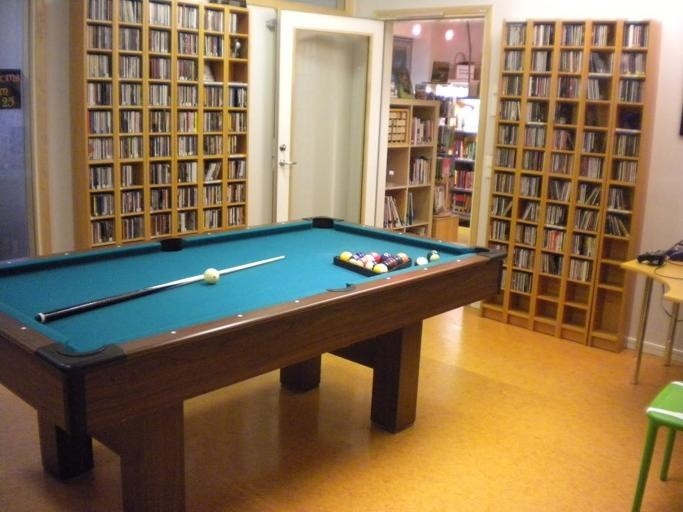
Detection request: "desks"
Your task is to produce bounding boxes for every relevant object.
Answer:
[2,217,507,511]
[619,259,683,383]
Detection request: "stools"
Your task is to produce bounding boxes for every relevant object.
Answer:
[632,381,682,510]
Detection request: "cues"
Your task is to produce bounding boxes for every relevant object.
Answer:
[35,255,285,325]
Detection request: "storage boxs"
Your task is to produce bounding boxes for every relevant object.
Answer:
[433,216,459,242]
[453,65,476,79]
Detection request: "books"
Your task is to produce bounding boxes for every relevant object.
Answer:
[501,270,533,293]
[501,75,643,124]
[495,123,640,183]
[86,1,245,244]
[491,172,633,238]
[382,108,480,240]
[490,222,596,281]
[504,22,649,74]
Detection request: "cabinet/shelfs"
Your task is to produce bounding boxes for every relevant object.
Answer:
[70,2,249,249]
[482,18,662,355]
[435,131,477,220]
[388,97,439,239]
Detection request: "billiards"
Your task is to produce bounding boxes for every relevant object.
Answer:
[414,256,428,266]
[427,250,439,262]
[203,268,218,284]
[339,250,408,273]
